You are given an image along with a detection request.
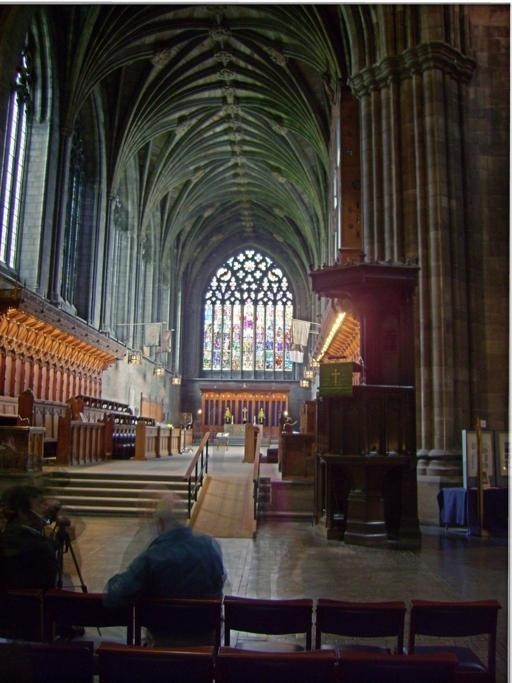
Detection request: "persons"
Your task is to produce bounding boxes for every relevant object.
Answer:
[2,484,82,645]
[103,489,228,645]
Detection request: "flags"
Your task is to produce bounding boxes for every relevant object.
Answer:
[144,322,161,346]
[289,327,305,353]
[154,329,172,353]
[292,318,310,346]
[288,350,305,363]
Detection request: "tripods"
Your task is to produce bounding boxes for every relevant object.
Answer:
[49,529,103,638]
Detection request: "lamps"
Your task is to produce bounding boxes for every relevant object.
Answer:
[299,359,320,388]
[129,353,182,385]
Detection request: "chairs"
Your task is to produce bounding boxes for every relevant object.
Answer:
[0,587,502,683]
